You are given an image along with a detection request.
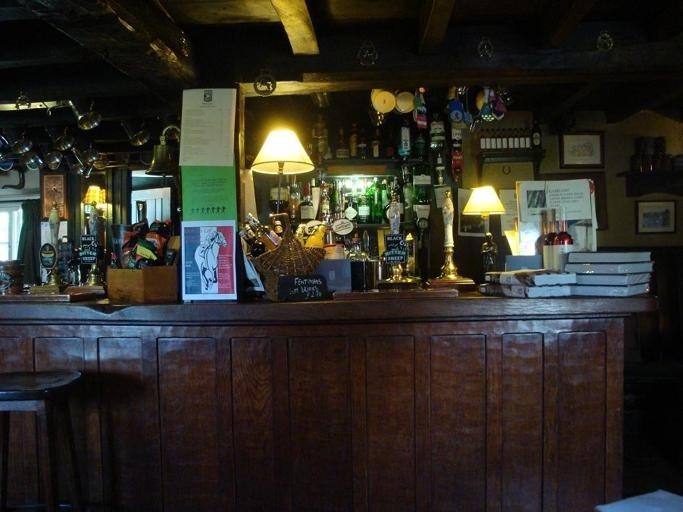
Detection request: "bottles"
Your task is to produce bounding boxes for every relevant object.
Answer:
[478,117,541,150]
[309,111,412,224]
[542,206,574,274]
[239,210,284,257]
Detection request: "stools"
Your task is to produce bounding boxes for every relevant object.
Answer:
[0,369,88,509]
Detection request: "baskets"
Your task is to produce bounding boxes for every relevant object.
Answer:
[250,224,326,302]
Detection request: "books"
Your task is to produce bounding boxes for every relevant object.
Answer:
[478,251,656,299]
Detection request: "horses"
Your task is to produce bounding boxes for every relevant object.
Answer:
[194,230,228,291]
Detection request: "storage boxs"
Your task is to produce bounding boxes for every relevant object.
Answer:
[103,264,178,305]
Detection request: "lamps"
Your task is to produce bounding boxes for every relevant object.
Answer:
[251,128,313,235]
[464,184,507,274]
[146,124,180,175]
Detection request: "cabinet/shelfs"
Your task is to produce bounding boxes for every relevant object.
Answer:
[241,90,423,232]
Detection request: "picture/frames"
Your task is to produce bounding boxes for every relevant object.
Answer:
[633,199,676,235]
[177,218,239,302]
[559,129,606,168]
